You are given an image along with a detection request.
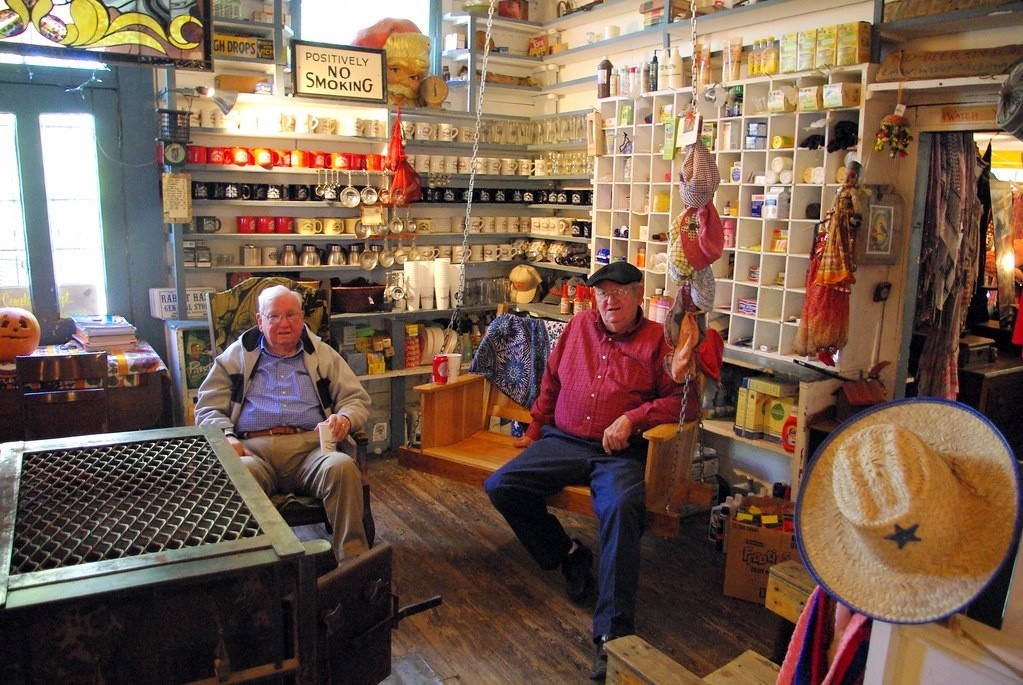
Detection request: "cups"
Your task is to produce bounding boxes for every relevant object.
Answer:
[604,26,620,40]
[444,353,462,383]
[317,421,339,452]
[586,32,594,44]
[170,105,598,312]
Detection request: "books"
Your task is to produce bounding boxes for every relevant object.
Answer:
[70,314,137,352]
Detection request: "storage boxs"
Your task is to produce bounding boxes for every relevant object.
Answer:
[691,442,717,480]
[814,25,838,70]
[680,476,719,518]
[733,388,750,436]
[823,82,860,109]
[836,20,872,67]
[723,497,804,603]
[779,33,796,74]
[743,376,799,397]
[767,91,795,113]
[746,136,764,149]
[744,390,771,440]
[763,395,798,445]
[748,122,767,138]
[799,86,824,111]
[796,28,817,71]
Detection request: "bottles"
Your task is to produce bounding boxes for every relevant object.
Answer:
[723,220,735,247]
[597,56,658,99]
[443,66,450,81]
[559,284,596,316]
[747,37,778,78]
[648,288,673,323]
[708,488,772,553]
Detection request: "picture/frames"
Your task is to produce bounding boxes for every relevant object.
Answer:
[289,39,388,104]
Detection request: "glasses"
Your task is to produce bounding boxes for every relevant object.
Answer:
[262,312,300,323]
[593,287,638,298]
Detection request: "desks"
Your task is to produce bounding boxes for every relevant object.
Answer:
[952,353,1023,420]
[0,341,175,445]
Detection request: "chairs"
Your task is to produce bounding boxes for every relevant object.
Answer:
[259,424,375,549]
[17,350,108,440]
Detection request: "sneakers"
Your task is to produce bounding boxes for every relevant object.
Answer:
[563,538,593,603]
[589,633,622,681]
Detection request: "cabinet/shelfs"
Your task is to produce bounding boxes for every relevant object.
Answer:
[589,61,876,387]
[425,0,883,114]
[155,66,597,450]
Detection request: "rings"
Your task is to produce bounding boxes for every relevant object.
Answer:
[342,424,346,427]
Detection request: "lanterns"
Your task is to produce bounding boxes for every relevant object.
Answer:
[0,307,41,364]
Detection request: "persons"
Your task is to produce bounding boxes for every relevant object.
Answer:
[352,17,431,107]
[484,262,700,680]
[194,284,372,567]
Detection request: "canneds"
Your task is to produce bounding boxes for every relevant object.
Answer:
[432,355,449,384]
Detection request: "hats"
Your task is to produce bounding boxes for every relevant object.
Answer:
[585,262,642,287]
[662,141,723,385]
[794,396,1023,625]
[508,264,586,305]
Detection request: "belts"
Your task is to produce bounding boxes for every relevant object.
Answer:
[240,426,307,439]
